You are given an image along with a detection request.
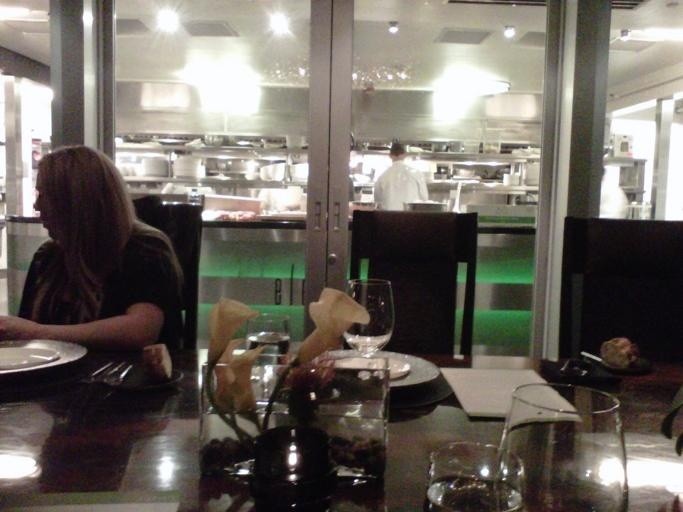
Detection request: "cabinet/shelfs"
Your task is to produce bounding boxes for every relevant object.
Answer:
[116,136,647,207]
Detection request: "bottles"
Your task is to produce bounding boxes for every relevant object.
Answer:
[187,188,203,207]
[621,136,629,152]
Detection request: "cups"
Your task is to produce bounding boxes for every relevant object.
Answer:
[500,383,630,511]
[627,205,641,219]
[423,442,525,512]
[504,174,518,186]
[249,428,336,511]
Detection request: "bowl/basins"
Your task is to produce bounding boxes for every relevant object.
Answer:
[140,156,201,176]
[257,162,308,183]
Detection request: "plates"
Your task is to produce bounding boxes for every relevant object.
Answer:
[75,365,182,392]
[600,355,652,375]
[314,351,440,386]
[541,360,619,381]
[0,340,91,377]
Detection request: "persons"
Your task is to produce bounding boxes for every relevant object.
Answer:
[0,141,187,357]
[370,139,428,211]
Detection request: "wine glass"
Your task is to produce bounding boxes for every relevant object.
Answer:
[247,311,293,405]
[342,277,396,368]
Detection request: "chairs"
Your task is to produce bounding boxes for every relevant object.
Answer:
[558,222,681,364]
[136,198,201,348]
[349,209,478,362]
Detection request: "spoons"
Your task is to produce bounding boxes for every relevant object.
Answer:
[80,359,133,387]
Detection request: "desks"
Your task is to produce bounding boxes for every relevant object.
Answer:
[1,341,680,510]
